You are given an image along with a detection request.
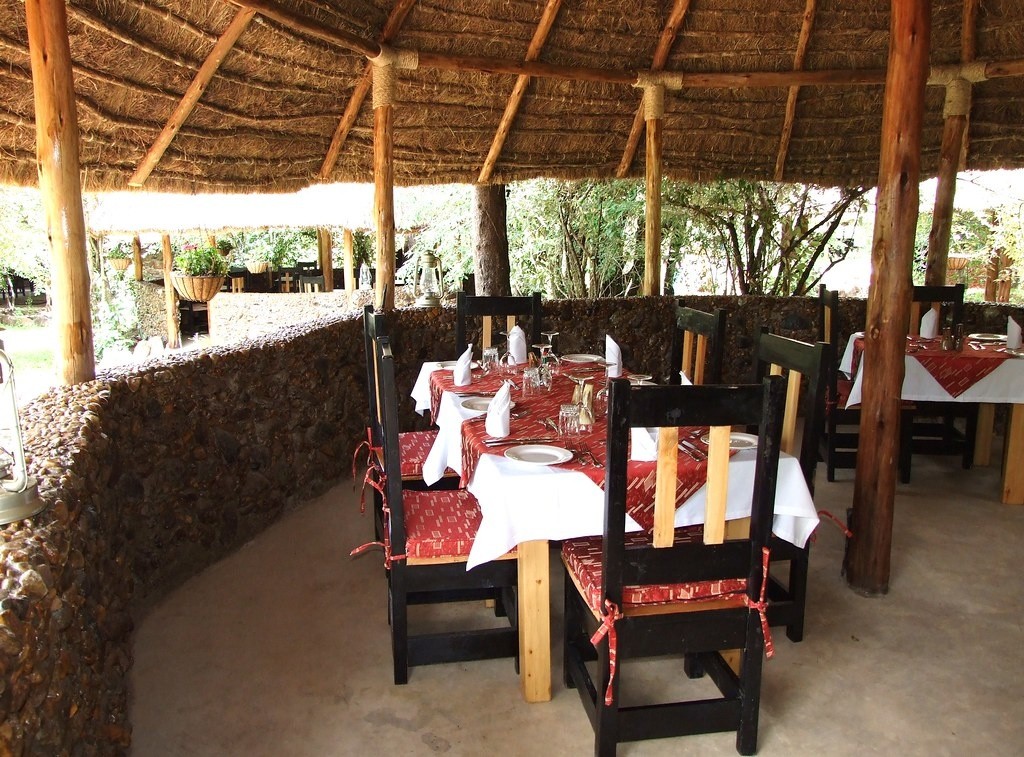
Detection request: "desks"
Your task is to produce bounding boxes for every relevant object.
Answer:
[838,331,1024,505]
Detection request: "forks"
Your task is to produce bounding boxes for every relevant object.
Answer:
[564,441,589,465]
[471,411,527,422]
[579,442,603,468]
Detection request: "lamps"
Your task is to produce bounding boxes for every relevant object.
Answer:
[0,348,47,526]
[413,249,443,307]
[358,262,372,289]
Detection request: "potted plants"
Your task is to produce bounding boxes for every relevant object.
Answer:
[169,244,229,301]
[243,251,270,273]
[106,242,131,270]
[216,239,233,255]
[946,244,969,270]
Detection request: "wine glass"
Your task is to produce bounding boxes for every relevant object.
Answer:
[498,332,517,378]
[540,331,559,376]
[532,344,552,392]
[627,375,652,389]
[567,373,593,435]
[596,364,620,416]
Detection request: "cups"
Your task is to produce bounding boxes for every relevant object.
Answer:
[482,347,498,376]
[559,405,580,438]
[523,367,540,398]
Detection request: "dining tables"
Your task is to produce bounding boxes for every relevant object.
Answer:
[410,358,820,702]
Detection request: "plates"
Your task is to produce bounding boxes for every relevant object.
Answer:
[461,397,515,412]
[701,432,758,449]
[504,444,574,465]
[437,361,478,370]
[968,334,1007,340]
[562,354,601,363]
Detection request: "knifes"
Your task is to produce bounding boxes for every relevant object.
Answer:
[487,440,553,448]
[482,437,553,444]
[681,440,708,458]
[678,444,700,461]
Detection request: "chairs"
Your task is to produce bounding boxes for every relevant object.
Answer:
[456,291,543,360]
[817,284,917,483]
[178,260,324,336]
[376,335,519,686]
[747,326,832,641]
[558,374,788,757]
[363,304,465,579]
[667,298,728,385]
[908,283,980,469]
